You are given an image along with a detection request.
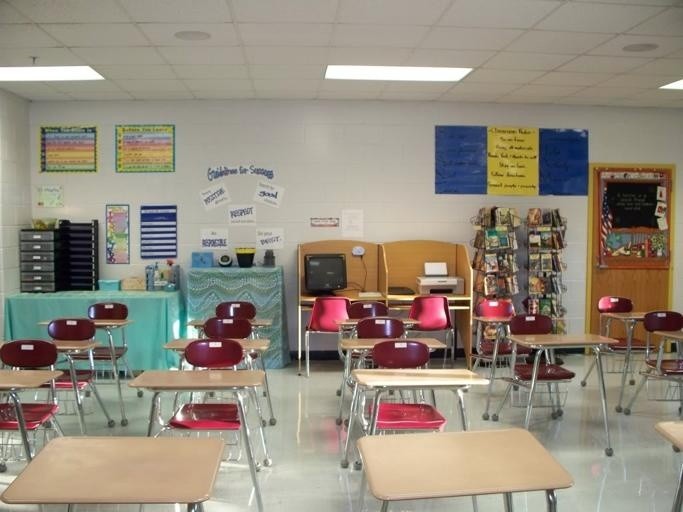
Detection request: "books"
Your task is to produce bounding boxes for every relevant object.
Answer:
[471,206,568,336]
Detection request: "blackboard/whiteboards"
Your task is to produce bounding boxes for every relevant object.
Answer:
[602,178,664,234]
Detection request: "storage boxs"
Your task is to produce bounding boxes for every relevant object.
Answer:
[98,280,120,291]
[145,264,180,291]
[121,279,146,291]
[32,217,57,229]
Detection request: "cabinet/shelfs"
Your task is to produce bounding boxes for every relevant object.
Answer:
[20,229,66,293]
[59,220,98,291]
[471,206,566,366]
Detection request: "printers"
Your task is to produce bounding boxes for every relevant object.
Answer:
[416,262,464,295]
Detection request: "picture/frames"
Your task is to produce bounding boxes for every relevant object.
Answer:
[38,124,97,172]
[115,124,175,173]
[106,205,130,264]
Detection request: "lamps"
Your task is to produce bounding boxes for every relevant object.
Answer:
[352,247,368,292]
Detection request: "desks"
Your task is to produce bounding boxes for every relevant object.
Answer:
[653,421,683,512]
[0,436,225,512]
[298,239,473,375]
[356,428,575,512]
[187,267,290,367]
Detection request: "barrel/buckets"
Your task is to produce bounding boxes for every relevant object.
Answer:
[235,247,256,267]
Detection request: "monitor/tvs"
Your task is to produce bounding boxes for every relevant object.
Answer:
[305,254,347,296]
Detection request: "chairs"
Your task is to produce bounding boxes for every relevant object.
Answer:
[0,295,683,471]
